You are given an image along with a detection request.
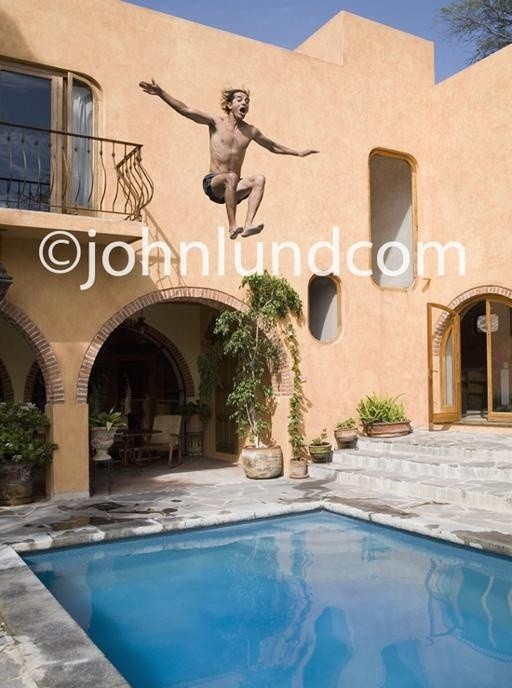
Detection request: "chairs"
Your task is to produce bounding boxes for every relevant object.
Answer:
[143,415,182,468]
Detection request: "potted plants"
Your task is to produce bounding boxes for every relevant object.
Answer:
[213,271,303,480]
[284,325,306,479]
[334,418,357,444]
[356,390,412,438]
[0,401,59,507]
[89,406,127,461]
[308,429,332,462]
[183,399,209,433]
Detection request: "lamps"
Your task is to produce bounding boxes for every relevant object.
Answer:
[135,309,146,344]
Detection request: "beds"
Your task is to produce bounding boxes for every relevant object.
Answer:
[89,461,112,497]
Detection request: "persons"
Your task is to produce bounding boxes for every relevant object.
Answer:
[138,77,320,240]
